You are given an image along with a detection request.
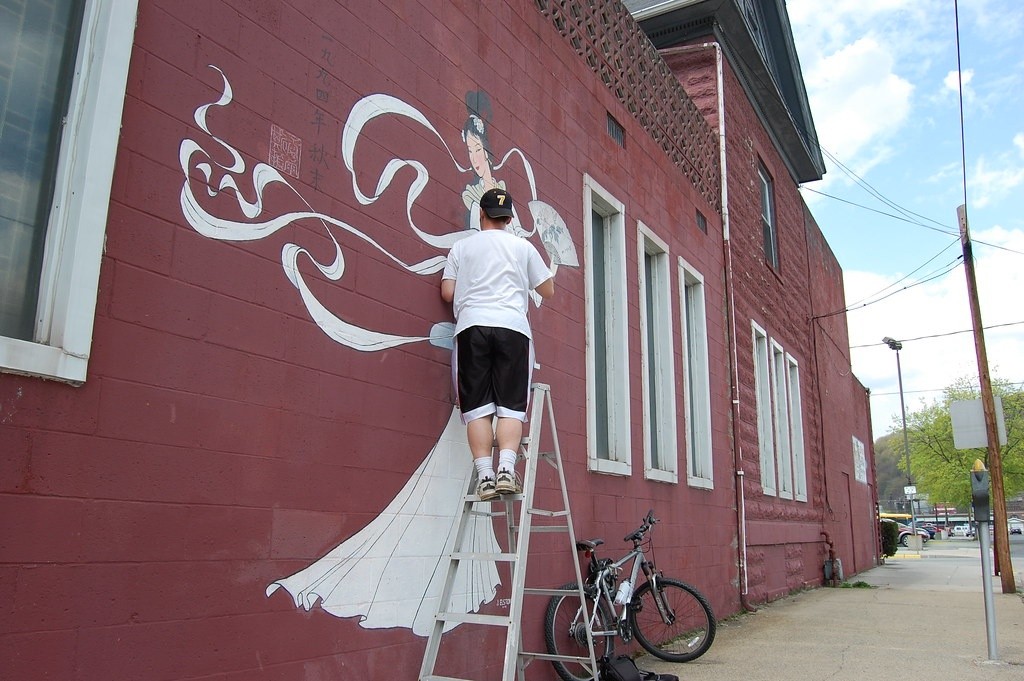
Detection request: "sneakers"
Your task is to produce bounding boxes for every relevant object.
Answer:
[495,467,522,494]
[476,476,499,502]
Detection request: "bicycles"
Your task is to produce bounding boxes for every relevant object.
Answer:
[543,509,717,681]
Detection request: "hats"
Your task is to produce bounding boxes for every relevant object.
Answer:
[480,188,515,218]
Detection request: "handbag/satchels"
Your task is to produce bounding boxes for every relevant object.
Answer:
[600,655,678,681]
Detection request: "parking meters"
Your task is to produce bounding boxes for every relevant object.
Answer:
[970,458,998,662]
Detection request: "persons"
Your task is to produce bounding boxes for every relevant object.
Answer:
[439,188,555,501]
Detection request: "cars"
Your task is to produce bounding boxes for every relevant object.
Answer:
[880,513,994,547]
[1010,525,1022,534]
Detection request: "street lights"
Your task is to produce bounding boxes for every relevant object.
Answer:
[882,337,917,538]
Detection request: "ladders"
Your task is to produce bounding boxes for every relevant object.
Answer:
[418,382,600,681]
